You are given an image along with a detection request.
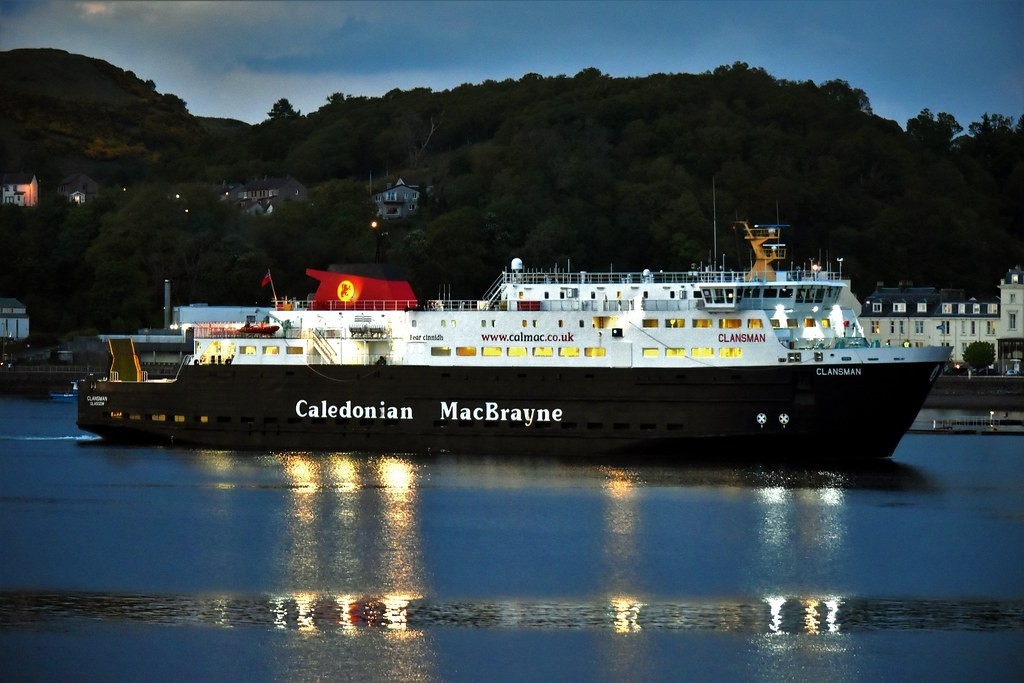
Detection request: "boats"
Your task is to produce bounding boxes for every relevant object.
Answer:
[242,324,278,334]
[77,220,953,470]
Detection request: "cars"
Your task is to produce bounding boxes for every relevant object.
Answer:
[1005,370,1021,375]
[952,367,996,375]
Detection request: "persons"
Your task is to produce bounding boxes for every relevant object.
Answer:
[903,339,911,348]
[886,339,890,346]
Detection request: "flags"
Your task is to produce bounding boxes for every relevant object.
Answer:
[262,273,271,286]
[936,325,944,330]
